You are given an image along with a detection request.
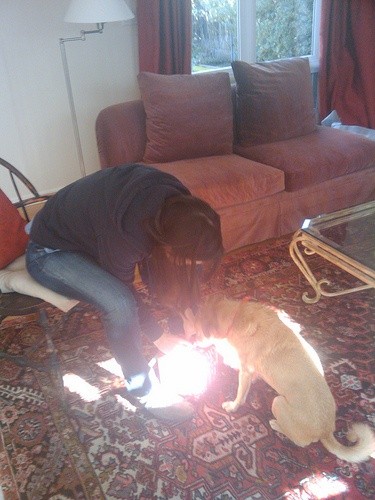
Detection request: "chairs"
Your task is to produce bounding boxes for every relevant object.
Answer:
[0,157,80,369]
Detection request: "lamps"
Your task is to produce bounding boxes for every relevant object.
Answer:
[59,0,134,178]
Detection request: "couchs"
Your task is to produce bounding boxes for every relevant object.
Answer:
[95,84,375,254]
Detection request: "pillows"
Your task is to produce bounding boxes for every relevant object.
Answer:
[137,69,234,163]
[232,56,317,147]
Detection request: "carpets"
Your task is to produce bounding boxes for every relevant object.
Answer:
[0,229,375,500]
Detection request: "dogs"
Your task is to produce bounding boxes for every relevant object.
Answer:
[191,291,375,465]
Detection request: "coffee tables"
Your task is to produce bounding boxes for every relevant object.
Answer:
[289,201,375,304]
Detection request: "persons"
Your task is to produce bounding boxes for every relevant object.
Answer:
[26,164,225,425]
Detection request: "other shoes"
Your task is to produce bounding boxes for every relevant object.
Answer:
[170,322,209,353]
[129,372,194,422]
